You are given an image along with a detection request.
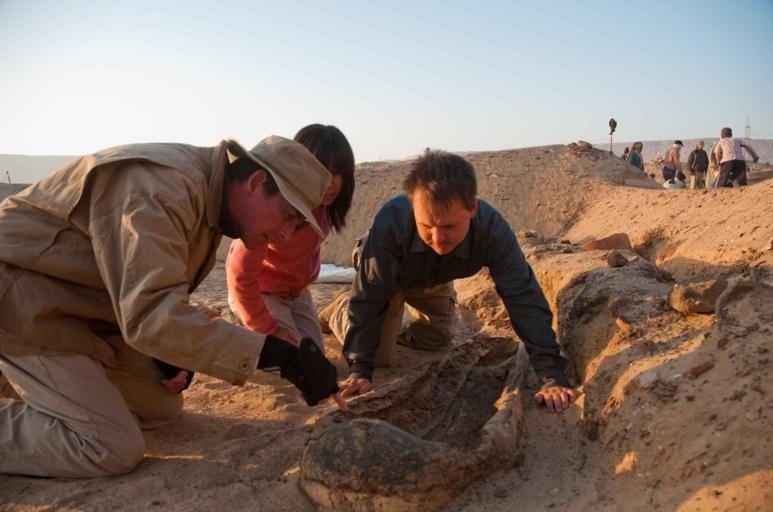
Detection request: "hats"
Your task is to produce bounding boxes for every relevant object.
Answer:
[227,136,331,238]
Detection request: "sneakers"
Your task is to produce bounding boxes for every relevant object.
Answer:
[317,289,351,334]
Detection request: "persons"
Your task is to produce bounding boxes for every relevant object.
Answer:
[688,140,710,190]
[314,152,575,415]
[627,141,644,171]
[620,146,630,160]
[662,139,686,188]
[0,131,350,478]
[714,128,759,188]
[222,124,354,365]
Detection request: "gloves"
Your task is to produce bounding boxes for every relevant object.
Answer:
[257,334,339,405]
[153,357,193,393]
